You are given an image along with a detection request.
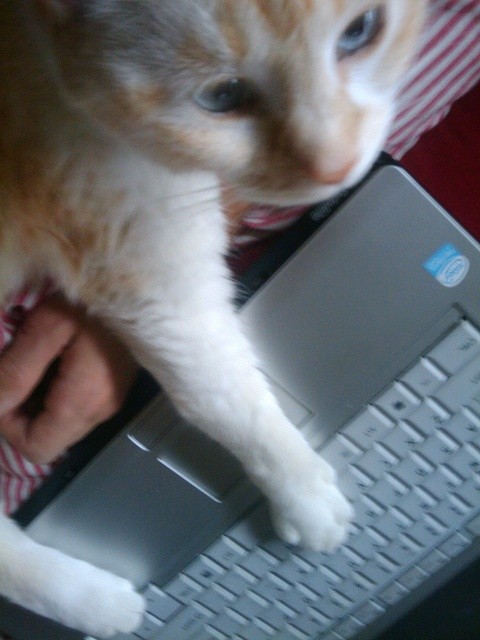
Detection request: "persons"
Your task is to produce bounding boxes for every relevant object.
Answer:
[0,292,137,466]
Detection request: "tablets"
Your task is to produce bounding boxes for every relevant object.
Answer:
[1,164,480,635]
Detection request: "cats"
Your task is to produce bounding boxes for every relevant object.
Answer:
[0,0,428,640]
